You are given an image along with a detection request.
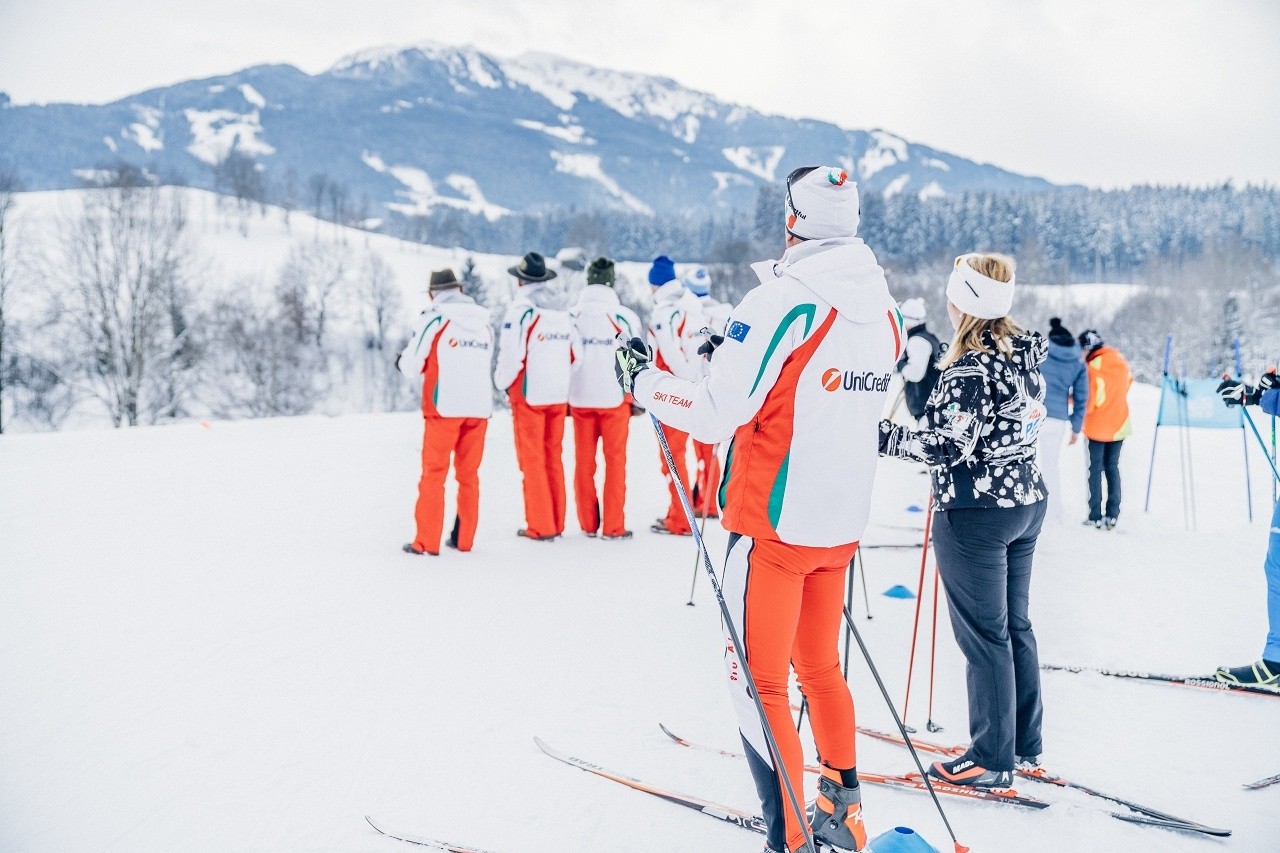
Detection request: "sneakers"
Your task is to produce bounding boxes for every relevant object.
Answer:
[403,543,438,555]
[444,539,456,548]
[693,511,720,519]
[1102,516,1117,530]
[586,531,598,537]
[517,530,554,542]
[1216,659,1280,691]
[928,755,1013,790]
[652,525,692,535]
[655,518,668,525]
[805,781,867,851]
[602,531,630,539]
[1015,754,1043,775]
[1083,518,1102,529]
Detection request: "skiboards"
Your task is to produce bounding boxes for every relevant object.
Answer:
[660,686,1231,842]
[854,518,933,548]
[1040,662,1279,789]
[364,736,767,853]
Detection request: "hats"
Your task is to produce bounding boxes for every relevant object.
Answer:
[587,258,615,286]
[422,268,464,289]
[507,252,557,281]
[784,166,860,239]
[683,268,712,296]
[900,298,926,319]
[1079,329,1103,349]
[649,256,676,285]
[1050,317,1074,345]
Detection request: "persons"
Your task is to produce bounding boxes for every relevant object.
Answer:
[490,250,586,545]
[613,163,908,853]
[892,296,946,550]
[1067,327,1135,531]
[1213,363,1280,693]
[679,267,737,525]
[867,249,1048,789]
[566,255,647,542]
[642,254,710,537]
[392,267,497,558]
[1034,313,1091,545]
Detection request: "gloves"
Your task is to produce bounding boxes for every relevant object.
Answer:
[1259,373,1278,390]
[615,336,654,394]
[877,420,911,458]
[698,336,723,362]
[1216,379,1261,408]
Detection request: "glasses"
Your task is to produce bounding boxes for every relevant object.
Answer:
[787,167,820,218]
